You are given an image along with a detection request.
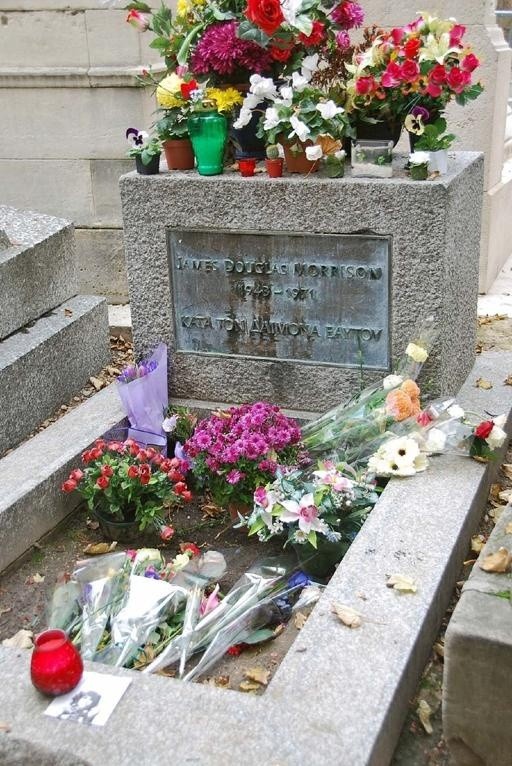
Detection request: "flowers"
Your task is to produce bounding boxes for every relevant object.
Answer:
[173,402,311,508]
[232,52,347,178]
[243,457,383,549]
[126,129,156,149]
[347,13,484,137]
[309,23,406,121]
[125,0,364,83]
[302,320,511,477]
[114,341,168,457]
[48,541,317,683]
[136,70,243,139]
[61,437,192,541]
[150,75,204,137]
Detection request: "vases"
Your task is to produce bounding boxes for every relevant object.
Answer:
[291,521,364,577]
[29,627,85,697]
[357,116,403,150]
[188,109,228,175]
[230,501,268,534]
[129,154,161,177]
[163,139,194,168]
[91,501,147,542]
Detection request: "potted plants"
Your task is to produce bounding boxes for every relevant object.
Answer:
[325,158,345,178]
[266,146,284,177]
[237,158,257,177]
[276,131,321,172]
[351,139,394,178]
[415,117,454,175]
